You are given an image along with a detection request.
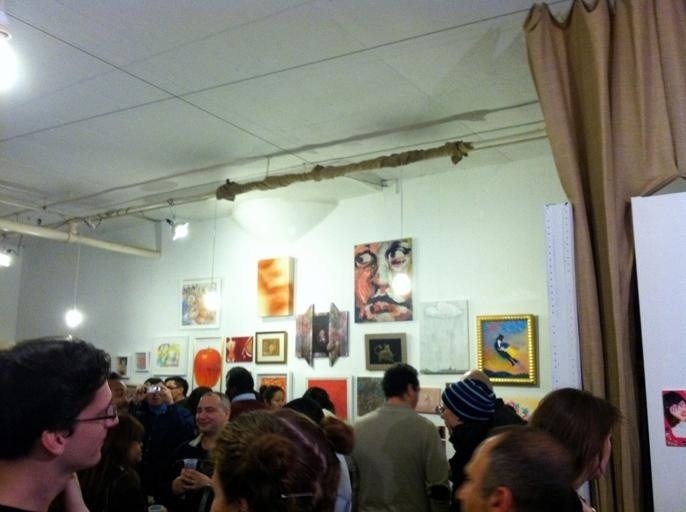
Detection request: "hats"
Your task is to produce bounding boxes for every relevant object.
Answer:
[441,379,495,424]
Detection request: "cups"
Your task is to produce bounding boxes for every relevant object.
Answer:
[148,504,162,512]
[182,458,198,471]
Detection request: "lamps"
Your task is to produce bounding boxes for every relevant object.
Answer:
[0,233,22,268]
[63,244,84,330]
[162,198,190,241]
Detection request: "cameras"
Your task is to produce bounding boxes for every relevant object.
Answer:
[147,386,161,393]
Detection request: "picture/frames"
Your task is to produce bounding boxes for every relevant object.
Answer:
[476,314,537,386]
[117,354,131,379]
[151,335,189,377]
[257,371,293,405]
[191,336,224,394]
[365,333,407,372]
[134,351,149,373]
[256,331,288,364]
[179,278,221,329]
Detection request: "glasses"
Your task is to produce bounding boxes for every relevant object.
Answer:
[74,405,117,423]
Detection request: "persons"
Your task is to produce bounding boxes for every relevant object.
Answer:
[457,425,574,512]
[528,387,620,512]
[343,364,451,512]
[0,335,119,512]
[355,241,413,322]
[66,336,354,511]
[436,370,528,512]
[663,391,686,438]
